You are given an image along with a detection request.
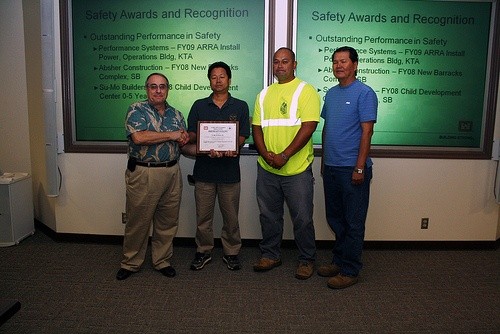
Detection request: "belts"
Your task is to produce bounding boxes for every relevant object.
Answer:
[137,161,178,167]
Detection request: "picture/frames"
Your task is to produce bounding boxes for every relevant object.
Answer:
[197,121,239,155]
[58,0,276,156]
[286,0,500,158]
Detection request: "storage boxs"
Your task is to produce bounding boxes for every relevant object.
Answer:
[0,172,35,247]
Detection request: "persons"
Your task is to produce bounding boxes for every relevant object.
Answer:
[177,62,250,270]
[116,73,223,280]
[318,46,378,289]
[252,47,321,279]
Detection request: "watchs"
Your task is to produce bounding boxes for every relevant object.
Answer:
[354,168,364,174]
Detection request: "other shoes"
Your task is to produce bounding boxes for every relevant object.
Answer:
[117,268,133,280]
[317,265,338,277]
[327,273,359,288]
[160,267,177,277]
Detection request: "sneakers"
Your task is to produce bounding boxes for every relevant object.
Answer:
[254,258,281,271]
[223,256,241,270]
[190,252,212,270]
[295,261,313,279]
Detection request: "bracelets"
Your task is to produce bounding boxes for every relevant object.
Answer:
[281,153,289,160]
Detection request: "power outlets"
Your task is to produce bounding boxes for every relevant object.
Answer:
[122,212,127,224]
[421,218,428,229]
